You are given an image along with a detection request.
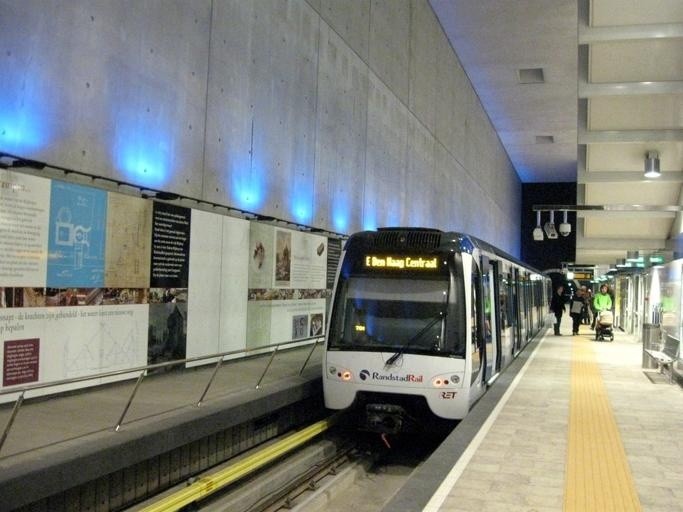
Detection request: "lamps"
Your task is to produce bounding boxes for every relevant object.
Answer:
[644,150,660,179]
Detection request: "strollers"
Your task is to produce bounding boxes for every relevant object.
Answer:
[595,310,615,342]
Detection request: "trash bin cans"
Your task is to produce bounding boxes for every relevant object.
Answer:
[643,323,662,368]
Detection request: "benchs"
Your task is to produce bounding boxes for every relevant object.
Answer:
[644,333,679,386]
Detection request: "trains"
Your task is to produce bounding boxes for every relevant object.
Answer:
[320,226,553,423]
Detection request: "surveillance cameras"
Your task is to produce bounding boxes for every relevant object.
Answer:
[545,223,558,239]
[560,224,571,236]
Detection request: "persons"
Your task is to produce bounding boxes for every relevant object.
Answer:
[592,283,613,313]
[550,284,566,336]
[580,284,589,324]
[585,287,594,326]
[589,296,598,329]
[659,284,678,314]
[569,287,586,335]
